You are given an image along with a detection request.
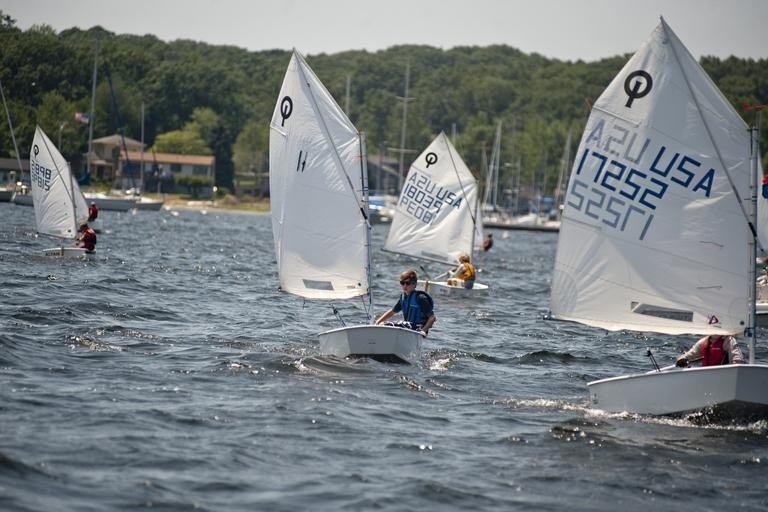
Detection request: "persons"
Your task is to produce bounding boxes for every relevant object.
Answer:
[87,202,99,223]
[674,335,746,367]
[447,253,476,290]
[478,232,494,252]
[74,223,98,252]
[373,268,436,338]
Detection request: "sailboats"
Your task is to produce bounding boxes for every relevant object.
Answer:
[269,48,425,364]
[544,15,768,418]
[380,130,490,298]
[367,63,570,233]
[2,42,165,255]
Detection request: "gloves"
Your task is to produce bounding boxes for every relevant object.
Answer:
[675,357,687,368]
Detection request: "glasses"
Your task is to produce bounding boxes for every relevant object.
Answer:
[400,280,412,285]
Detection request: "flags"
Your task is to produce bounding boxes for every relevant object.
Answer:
[75,112,90,124]
